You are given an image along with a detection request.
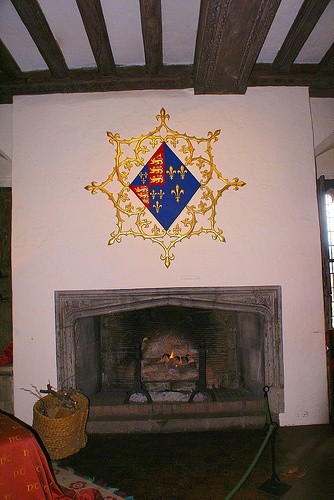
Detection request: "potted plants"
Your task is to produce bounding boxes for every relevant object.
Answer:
[19,374,89,460]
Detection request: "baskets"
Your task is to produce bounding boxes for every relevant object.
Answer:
[33,391,89,460]
